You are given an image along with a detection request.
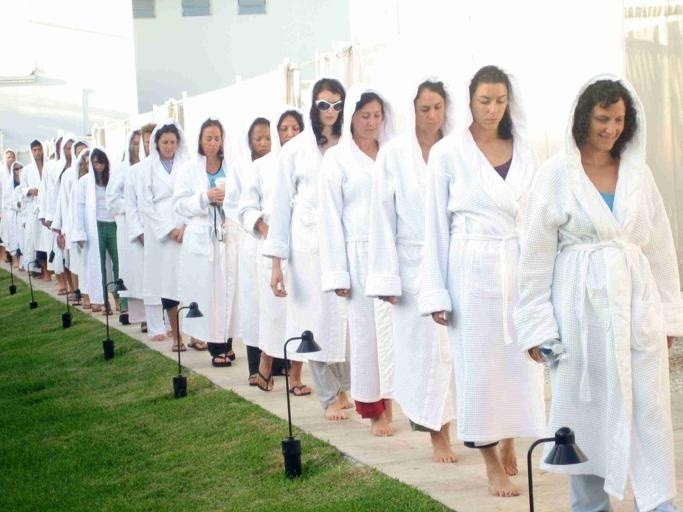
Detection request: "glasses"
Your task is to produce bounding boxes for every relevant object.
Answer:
[315,99,343,113]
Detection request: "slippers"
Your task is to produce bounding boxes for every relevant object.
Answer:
[248,376,257,385]
[188,341,208,350]
[289,385,311,396]
[172,344,186,352]
[57,288,66,295]
[103,309,113,315]
[226,351,235,361]
[212,355,231,367]
[257,354,272,391]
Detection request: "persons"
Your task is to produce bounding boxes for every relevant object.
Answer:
[105,129,141,326]
[510,72,683,512]
[365,74,460,464]
[51,138,74,296]
[4,160,25,269]
[125,122,158,333]
[71,146,118,317]
[51,140,91,310]
[135,121,186,343]
[262,77,354,420]
[239,106,313,396]
[222,114,293,388]
[64,148,102,313]
[417,64,547,498]
[0,148,16,263]
[173,118,237,368]
[38,135,62,281]
[19,139,51,282]
[320,82,393,438]
